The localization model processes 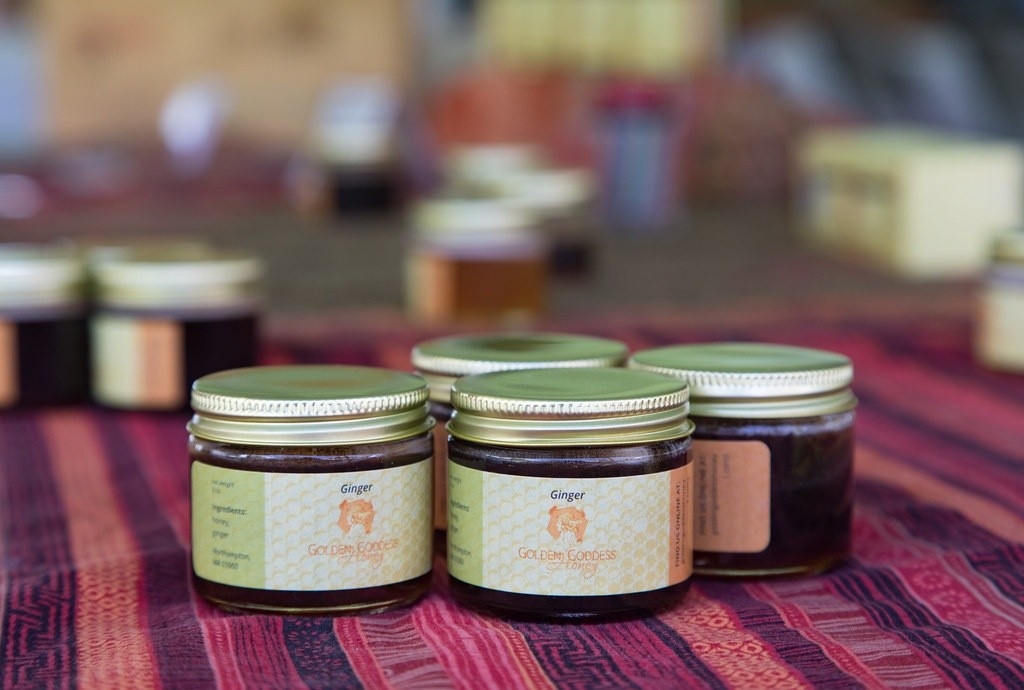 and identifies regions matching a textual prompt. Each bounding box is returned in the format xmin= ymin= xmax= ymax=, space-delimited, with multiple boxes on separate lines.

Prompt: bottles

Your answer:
xmin=409 ymin=200 xmax=541 ymax=334
xmin=409 ymin=331 xmax=630 ymax=545
xmin=1 ymin=245 xmax=85 ymax=411
xmin=970 ymin=239 xmax=1024 ymax=372
xmin=625 ymin=339 xmax=857 ymax=582
xmin=85 ymin=253 xmax=268 ymax=412
xmin=447 ymin=366 xmax=697 ymax=625
xmin=185 ymin=366 xmax=438 ymax=617
xmin=591 ymin=80 xmax=671 ymax=234
